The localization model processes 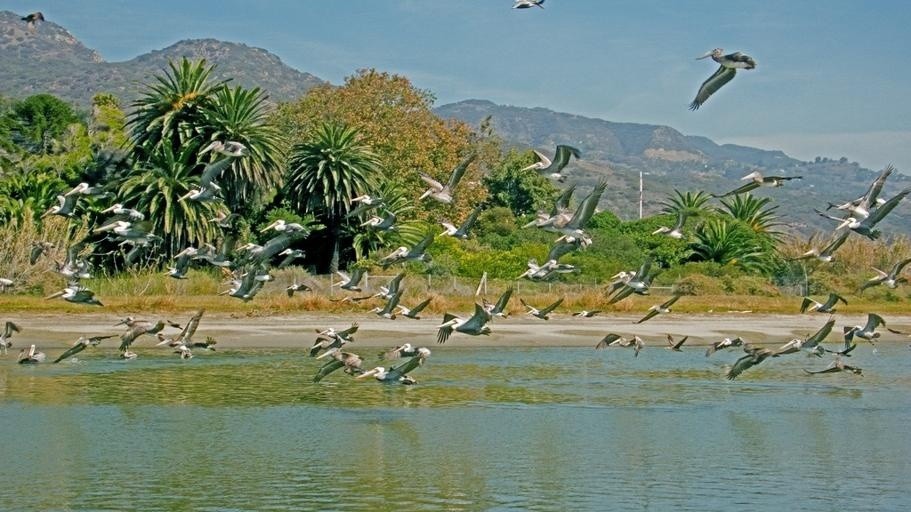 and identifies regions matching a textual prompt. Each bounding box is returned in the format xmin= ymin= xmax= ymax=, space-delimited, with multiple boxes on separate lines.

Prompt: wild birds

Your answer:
xmin=695 ymin=135 xmax=910 ymax=391
xmin=18 ymin=11 xmax=47 ymax=28
xmin=1 ymin=136 xmax=312 ymax=390
xmin=313 ymin=138 xmax=695 ymax=390
xmin=687 ymin=45 xmax=755 ymax=112
xmin=510 ymin=1 xmax=549 ymax=13
